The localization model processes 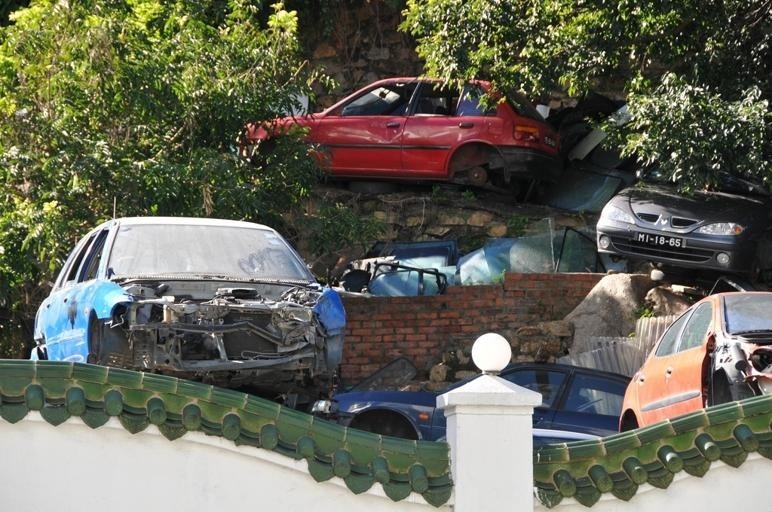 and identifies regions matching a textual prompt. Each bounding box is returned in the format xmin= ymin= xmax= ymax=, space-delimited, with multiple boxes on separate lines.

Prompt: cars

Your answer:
xmin=311 ymin=360 xmax=631 ymax=448
xmin=620 ymin=292 xmax=772 ymax=430
xmin=231 ymin=77 xmax=567 ymax=198
xmin=594 ymin=165 xmax=772 ymax=279
xmin=29 ymin=216 xmax=344 ymax=397
